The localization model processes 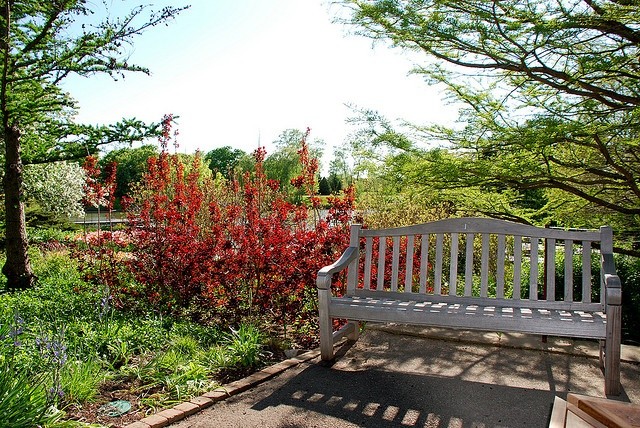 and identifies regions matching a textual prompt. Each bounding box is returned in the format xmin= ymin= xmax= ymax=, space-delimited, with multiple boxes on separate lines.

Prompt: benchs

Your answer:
xmin=314 ymin=217 xmax=625 ymax=396
